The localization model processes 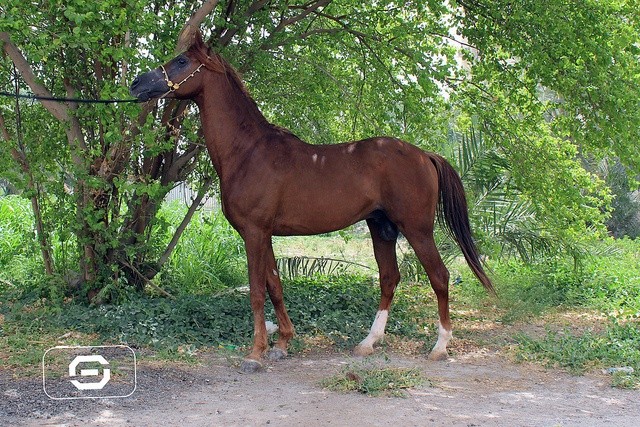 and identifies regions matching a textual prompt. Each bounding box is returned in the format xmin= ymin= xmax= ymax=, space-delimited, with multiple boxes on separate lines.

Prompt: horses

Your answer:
xmin=131 ymin=30 xmax=500 ymax=375
